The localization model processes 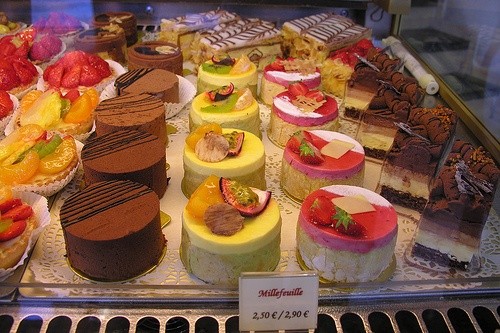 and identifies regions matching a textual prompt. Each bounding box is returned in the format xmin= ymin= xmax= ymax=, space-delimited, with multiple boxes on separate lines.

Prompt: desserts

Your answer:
xmin=0 ymin=9 xmax=500 ymax=286
